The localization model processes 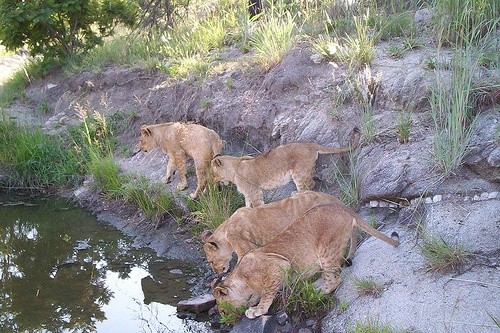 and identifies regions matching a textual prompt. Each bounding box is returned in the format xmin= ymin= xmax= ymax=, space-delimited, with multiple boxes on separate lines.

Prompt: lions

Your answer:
xmin=138 ymin=121 xmax=223 ymax=201
xmin=200 ymin=190 xmax=401 ymax=276
xmin=211 ymin=203 xmax=358 ymax=326
xmin=210 ymin=126 xmax=361 ymax=209
